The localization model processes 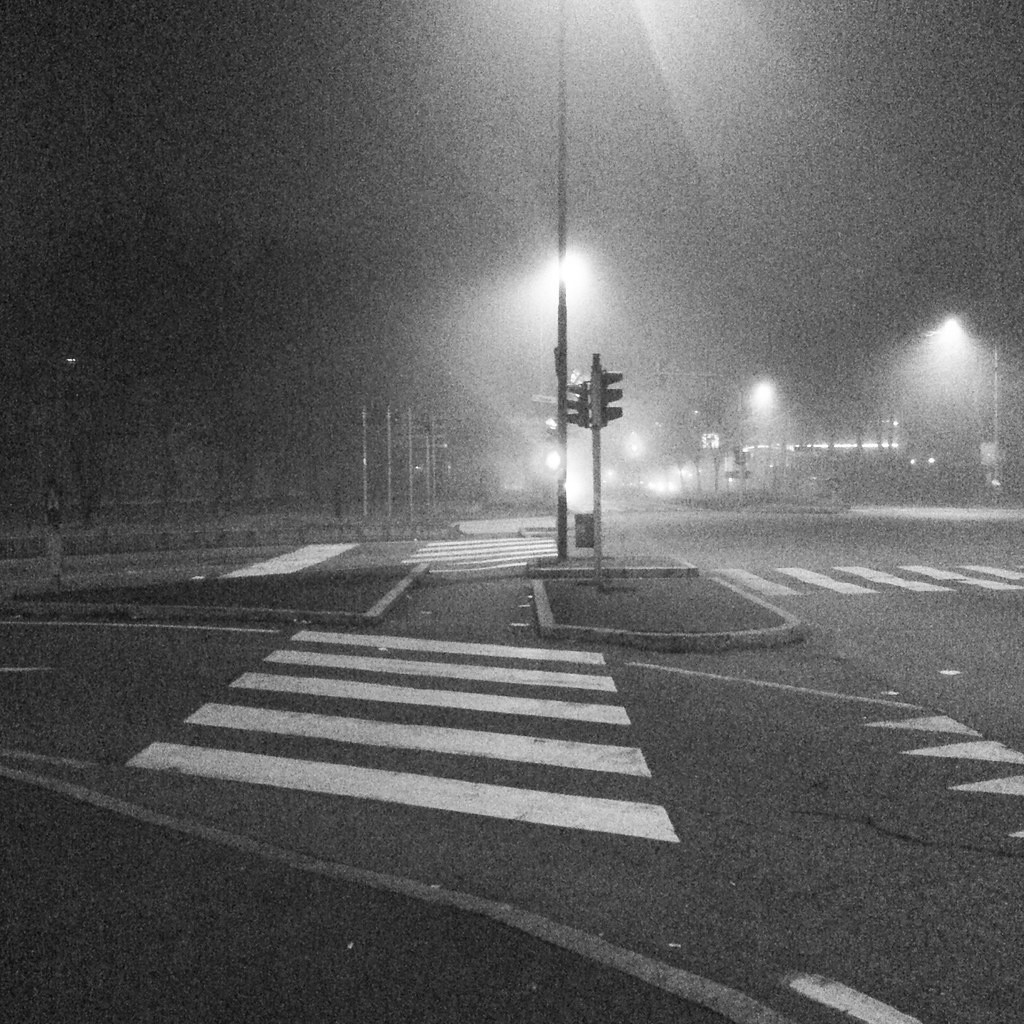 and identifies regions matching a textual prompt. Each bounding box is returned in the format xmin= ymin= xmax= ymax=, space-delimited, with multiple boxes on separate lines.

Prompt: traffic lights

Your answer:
xmin=590 ymin=367 xmax=624 ymax=429
xmin=566 ymin=382 xmax=591 ymax=429
